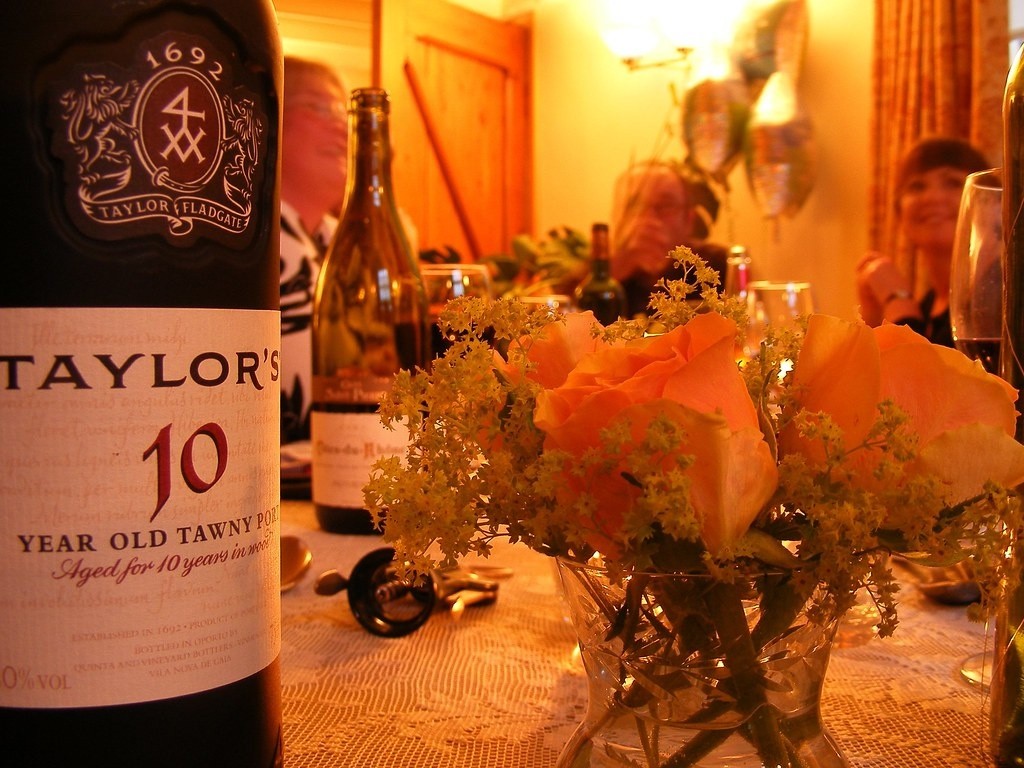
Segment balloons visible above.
[676,0,826,228]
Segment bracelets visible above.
[876,287,917,311]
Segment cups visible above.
[740,281,821,399]
[417,264,495,359]
[511,295,576,345]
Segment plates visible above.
[893,555,997,604]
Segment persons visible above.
[267,51,353,451]
[553,156,739,317]
[849,135,1003,378]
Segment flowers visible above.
[364,243,1024,768]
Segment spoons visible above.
[274,533,312,594]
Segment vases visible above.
[554,540,857,768]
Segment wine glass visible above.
[948,165,1007,689]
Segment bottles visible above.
[718,244,752,298]
[0,0,285,768]
[310,86,431,537]
[573,224,627,325]
[989,45,1024,768]
